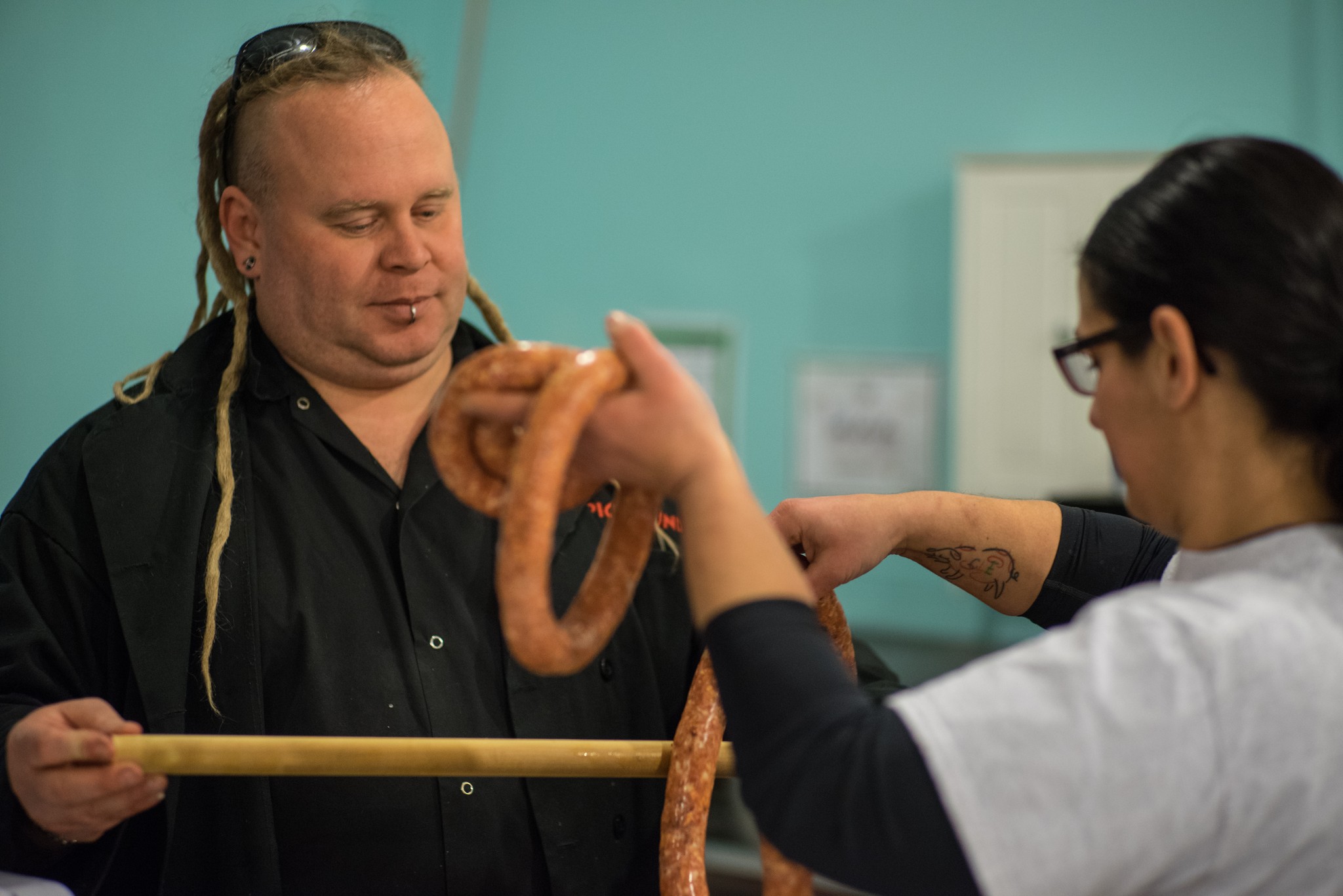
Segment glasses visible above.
[1053,317,1216,396]
[222,21,407,186]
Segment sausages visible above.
[432,340,858,896]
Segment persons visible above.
[0,16,902,896]
[461,136,1343,896]
